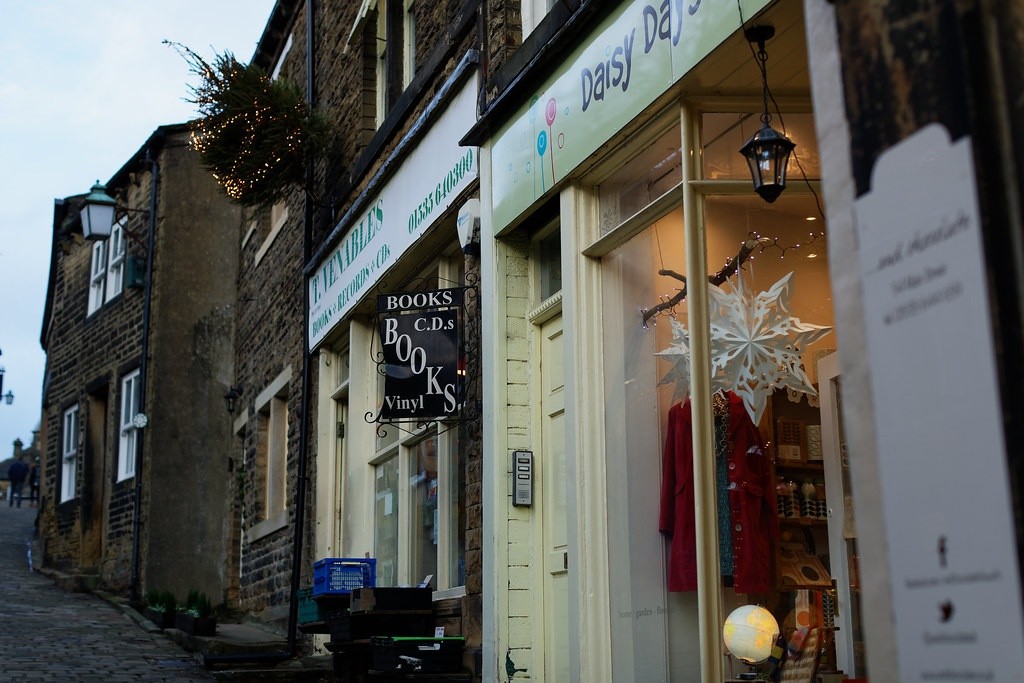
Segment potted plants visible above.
[144,585,216,636]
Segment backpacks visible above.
[34,468,40,486]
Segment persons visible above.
[8,456,41,508]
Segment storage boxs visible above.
[296,558,466,674]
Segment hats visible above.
[35,456,40,461]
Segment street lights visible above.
[78,180,156,601]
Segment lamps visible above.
[224,383,242,416]
[738,24,797,203]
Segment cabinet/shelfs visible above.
[748,383,861,617]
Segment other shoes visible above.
[9,501,14,507]
[17,502,21,508]
[29,502,33,507]
[36,503,40,508]
